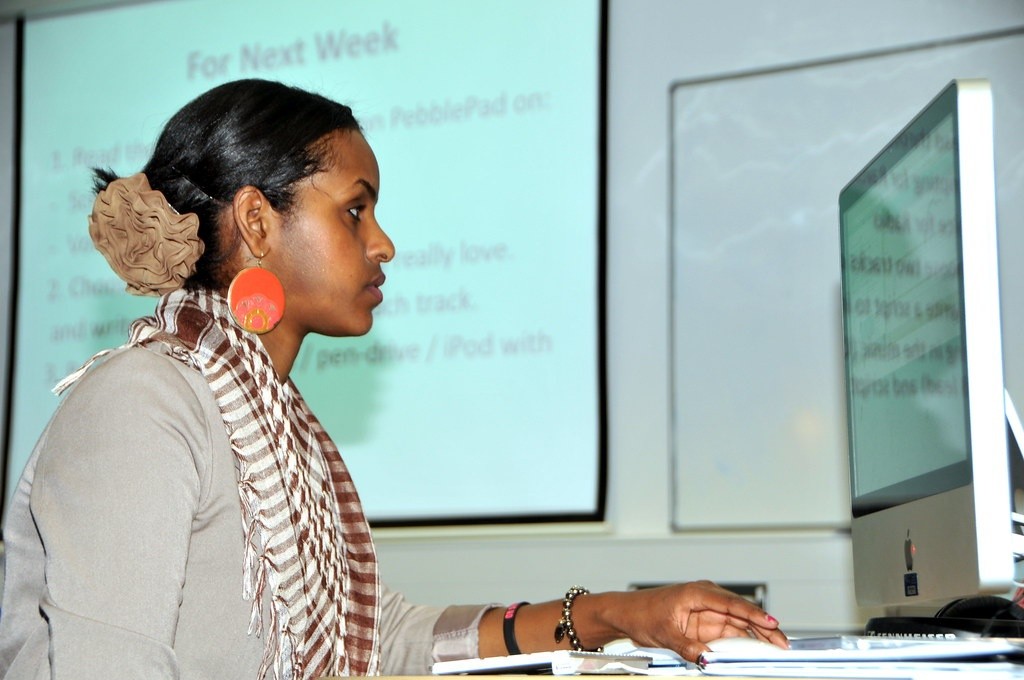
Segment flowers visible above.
[86,173,206,298]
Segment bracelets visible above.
[554,586,603,653]
[503,601,531,655]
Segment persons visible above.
[0,79,789,680]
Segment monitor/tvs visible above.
[838,78,1024,609]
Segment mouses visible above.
[685,637,787,670]
